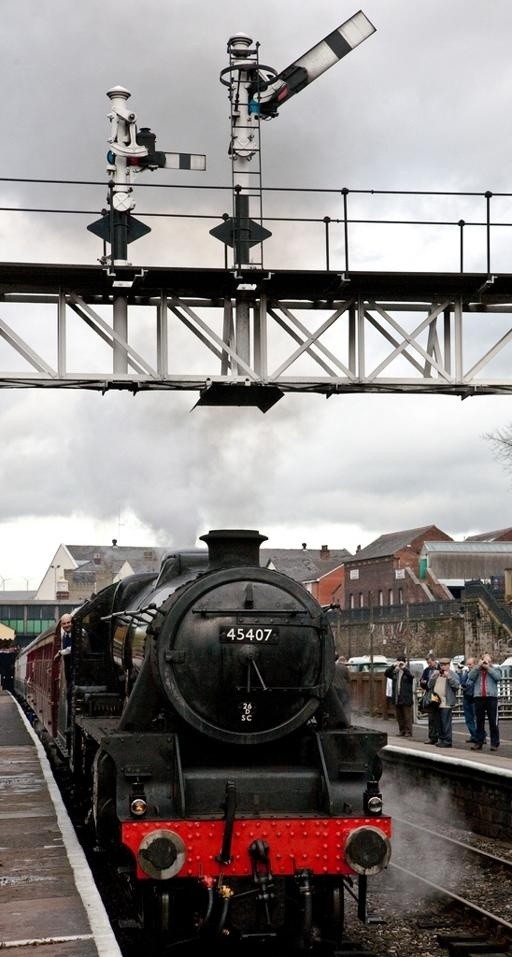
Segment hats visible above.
[440,658,450,663]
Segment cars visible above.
[337,651,512,703]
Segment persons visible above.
[456,656,478,743]
[335,655,352,726]
[427,657,459,748]
[54,611,90,734]
[418,653,442,745]
[466,652,503,751]
[383,654,414,737]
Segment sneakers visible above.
[406,733,412,737]
[396,733,405,736]
[490,746,497,751]
[466,739,476,743]
[471,745,482,750]
[424,739,438,745]
[436,742,452,748]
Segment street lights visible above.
[50,565,62,598]
[11,523,392,955]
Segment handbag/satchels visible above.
[418,692,441,714]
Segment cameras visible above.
[439,670,445,677]
[399,663,403,668]
[480,661,487,672]
